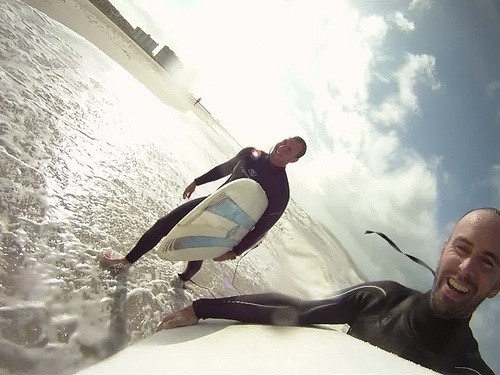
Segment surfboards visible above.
[157,178,269,262]
[72,318,442,375]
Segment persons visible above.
[156,207,500,375]
[99,135,307,290]
